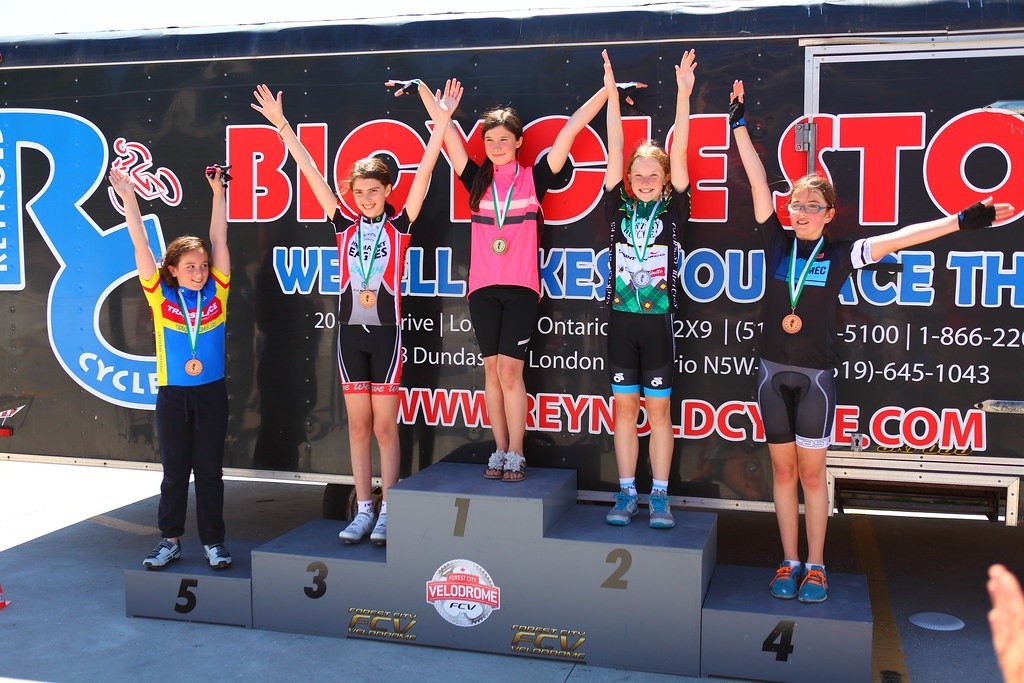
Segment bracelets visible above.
[277,121,289,143]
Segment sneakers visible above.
[203,543,232,566]
[798,565,828,601]
[769,560,802,598]
[338,513,374,542]
[143,538,182,568]
[370,513,387,541]
[605,488,638,525]
[648,491,674,527]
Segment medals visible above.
[781,316,803,333]
[632,270,650,288]
[185,359,202,376]
[491,238,507,255]
[359,291,376,308]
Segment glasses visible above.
[788,203,830,214]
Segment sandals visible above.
[483,449,507,478]
[502,450,526,481]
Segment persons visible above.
[251,77,463,548]
[727,80,1017,598]
[384,79,648,478]
[106,166,231,570]
[978,565,1024,683]
[600,47,697,532]
